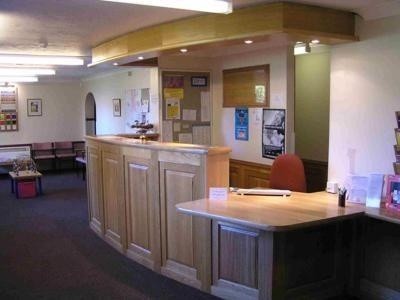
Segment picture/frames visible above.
[112,99,121,117]
[27,99,42,116]
[191,76,208,86]
[386,174,400,212]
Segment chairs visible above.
[31,141,86,180]
[269,153,307,192]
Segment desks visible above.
[360,202,400,300]
[174,187,364,300]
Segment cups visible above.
[338,188,346,208]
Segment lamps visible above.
[293,42,311,55]
[103,0,234,15]
[0,55,84,91]
